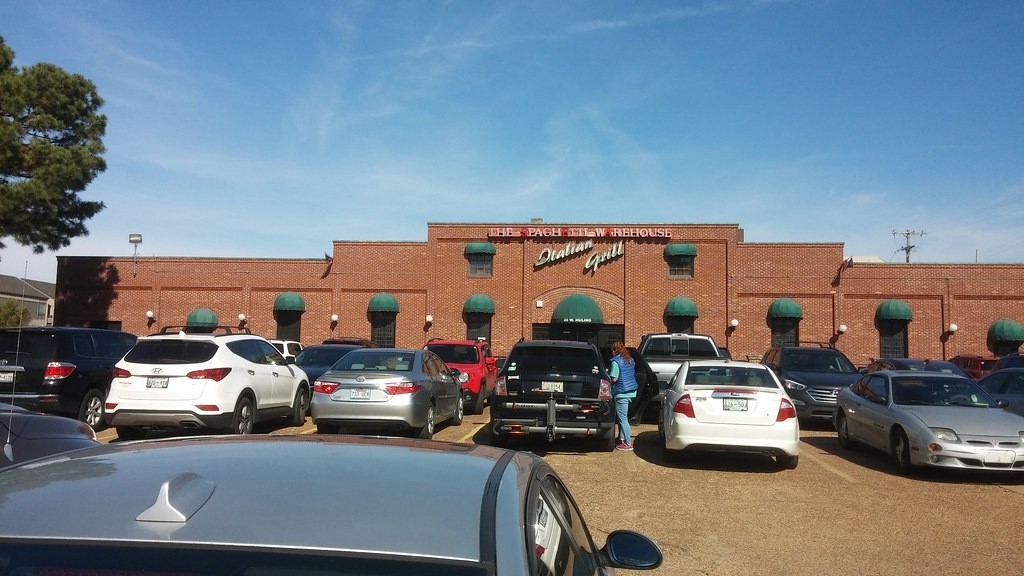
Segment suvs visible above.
[105,324,311,444]
[489,337,660,452]
[758,341,869,424]
[416,338,498,415]
[0,325,140,433]
[266,338,385,400]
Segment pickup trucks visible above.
[634,332,732,405]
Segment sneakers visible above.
[615,437,626,444]
[615,442,634,451]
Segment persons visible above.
[610,342,639,451]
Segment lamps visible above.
[146,310,156,322]
[238,313,247,324]
[837,324,848,335]
[331,314,339,321]
[949,323,957,333]
[425,315,433,323]
[731,319,739,330]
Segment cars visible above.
[832,369,1024,476]
[861,352,1024,413]
[657,359,800,469]
[310,347,466,440]
[1,403,665,575]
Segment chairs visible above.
[695,374,711,384]
[351,363,365,369]
[747,376,762,386]
[650,340,664,353]
[395,364,409,370]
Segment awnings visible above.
[665,296,698,318]
[464,243,496,255]
[368,292,400,313]
[876,299,912,321]
[274,291,305,312]
[551,294,604,324]
[463,294,495,314]
[989,320,1024,342]
[186,308,218,328]
[768,298,803,320]
[664,244,697,257]
